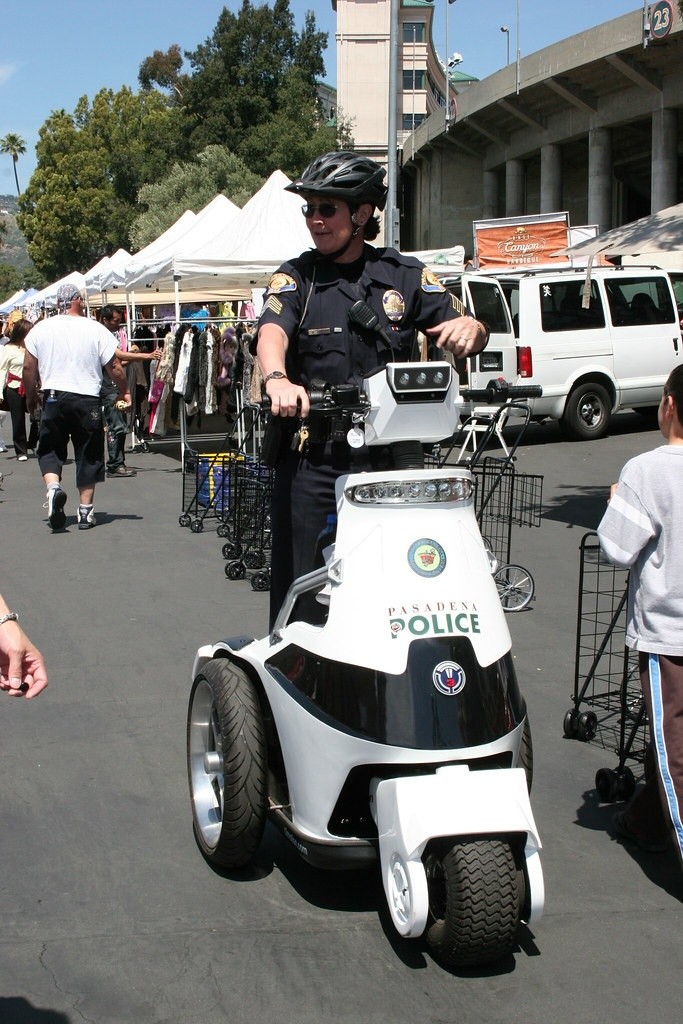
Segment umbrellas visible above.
[548,202,683,308]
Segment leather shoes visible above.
[107,466,137,478]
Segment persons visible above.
[99,304,163,478]
[21,283,132,534]
[249,152,490,632]
[597,364,683,868]
[0,319,40,461]
[0,594,48,699]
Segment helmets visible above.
[284,151,389,212]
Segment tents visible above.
[125,168,470,475]
[0,168,242,350]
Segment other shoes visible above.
[17,453,27,461]
[613,810,667,852]
[32,447,38,457]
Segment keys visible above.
[291,430,309,454]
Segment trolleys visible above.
[561,529,652,804]
[177,402,538,615]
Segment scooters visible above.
[183,358,548,975]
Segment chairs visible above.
[561,293,664,325]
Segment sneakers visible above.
[42,487,67,533]
[77,504,96,529]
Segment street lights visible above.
[500,26,510,65]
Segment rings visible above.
[30,407,33,409]
[460,337,467,341]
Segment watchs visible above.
[264,371,287,384]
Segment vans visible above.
[426,262,683,441]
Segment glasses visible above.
[301,201,361,218]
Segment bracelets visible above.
[123,393,129,394]
[471,318,483,335]
[0,613,18,625]
